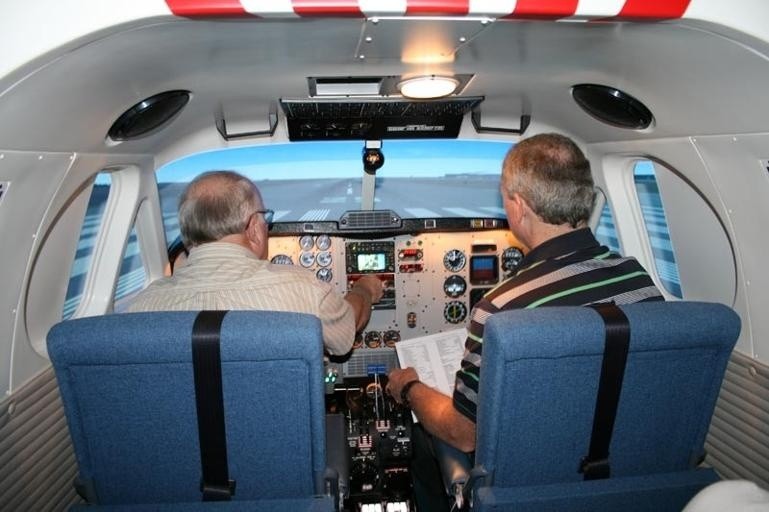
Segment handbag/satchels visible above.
[350,283,374,304]
[399,379,420,408]
[388,326,470,425]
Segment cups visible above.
[244,207,275,232]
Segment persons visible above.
[121,169,387,362]
[381,132,669,464]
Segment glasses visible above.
[394,76,459,99]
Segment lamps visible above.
[430,301,741,512]
[47,310,351,512]
